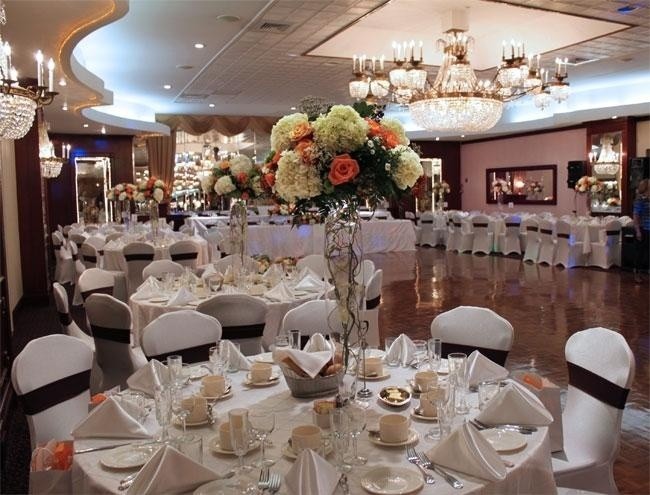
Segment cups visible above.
[121,392,144,426]
[153,263,300,299]
[479,379,502,410]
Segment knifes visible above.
[74,443,129,454]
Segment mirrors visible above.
[590,130,623,214]
[485,164,557,205]
[74,155,112,225]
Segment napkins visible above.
[381,331,419,366]
[283,446,343,493]
[466,347,510,386]
[68,393,158,440]
[123,442,220,495]
[216,338,253,374]
[474,378,555,427]
[130,266,330,306]
[426,423,509,486]
[126,357,172,398]
[301,331,332,352]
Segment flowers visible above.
[102,184,137,202]
[196,151,272,214]
[574,175,601,195]
[525,176,545,193]
[431,182,451,202]
[131,175,172,207]
[261,90,426,231]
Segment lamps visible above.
[344,5,571,138]
[0,0,59,139]
[590,131,622,177]
[36,100,72,181]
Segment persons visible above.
[633,179,650,284]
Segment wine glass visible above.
[145,328,474,475]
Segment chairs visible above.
[77,267,116,393]
[123,241,155,302]
[139,306,224,367]
[276,297,353,350]
[82,291,147,402]
[193,293,269,355]
[429,303,516,372]
[67,240,89,307]
[49,281,97,353]
[46,205,639,284]
[10,336,95,492]
[351,257,385,351]
[547,325,638,493]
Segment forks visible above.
[401,442,464,490]
[255,464,282,495]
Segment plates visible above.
[98,446,155,470]
[191,475,251,495]
[363,467,423,495]
[291,291,308,296]
[476,429,527,454]
